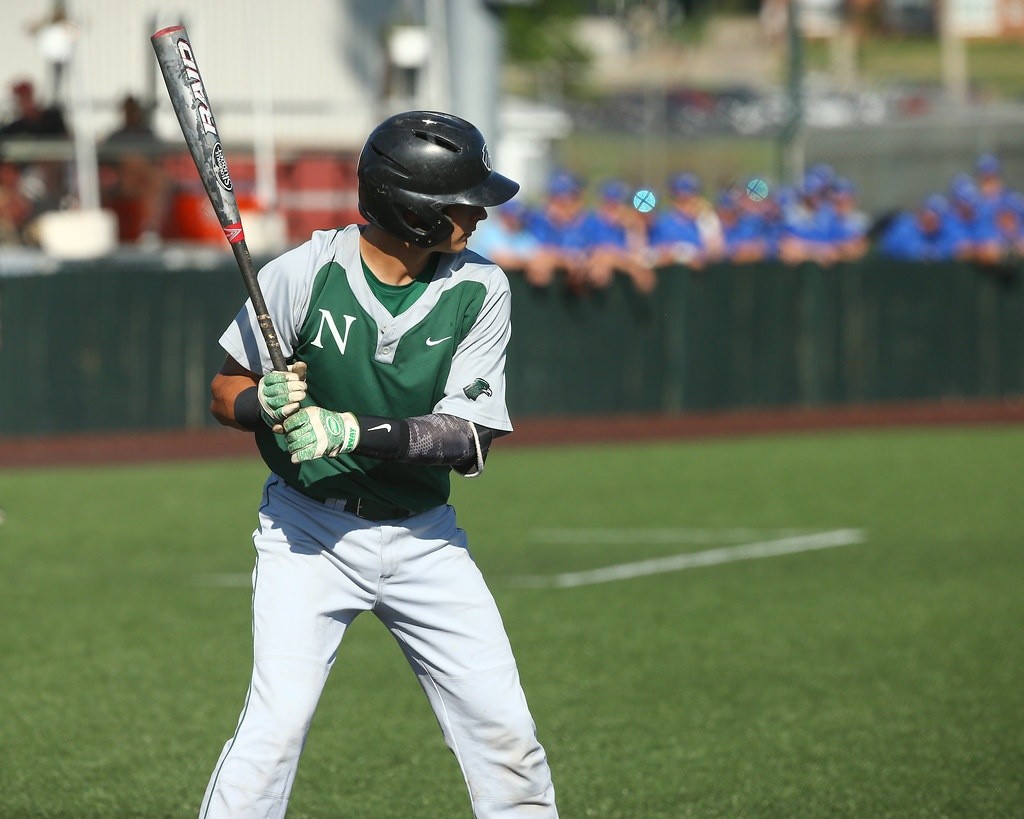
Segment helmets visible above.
[358,111,520,248]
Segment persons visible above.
[468,151,1024,298]
[197,109,560,819]
[0,83,169,237]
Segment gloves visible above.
[273,406,361,464]
[258,362,307,427]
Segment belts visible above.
[286,481,409,518]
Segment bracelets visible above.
[350,411,410,463]
[233,384,261,433]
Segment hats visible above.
[926,195,950,214]
[549,172,579,194]
[803,173,825,195]
[776,188,799,208]
[835,175,854,192]
[669,174,698,192]
[977,156,1002,173]
[602,180,629,200]
[953,176,977,204]
[815,165,835,184]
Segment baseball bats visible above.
[149,25,288,373]
